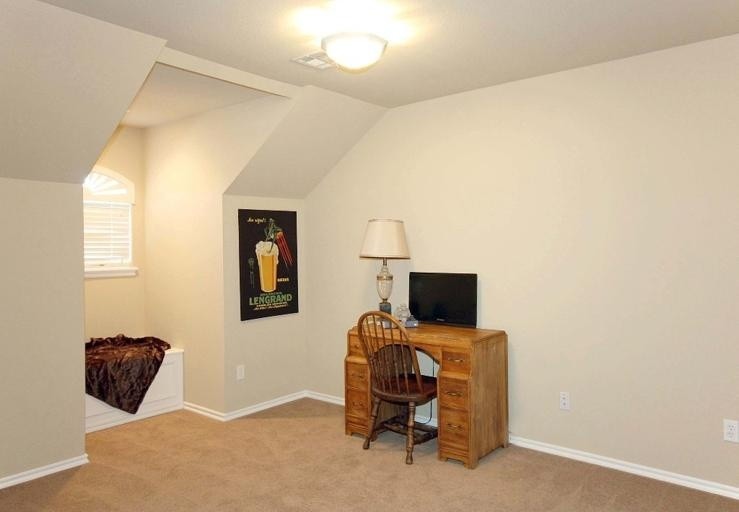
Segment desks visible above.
[344,328,509,470]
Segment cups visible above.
[255,242,278,292]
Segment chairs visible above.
[357,311,437,464]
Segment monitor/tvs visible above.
[408,271,477,329]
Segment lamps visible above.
[359,218,411,329]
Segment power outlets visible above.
[723,418,739,443]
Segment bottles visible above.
[393,304,416,323]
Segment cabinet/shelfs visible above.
[85,348,185,438]
